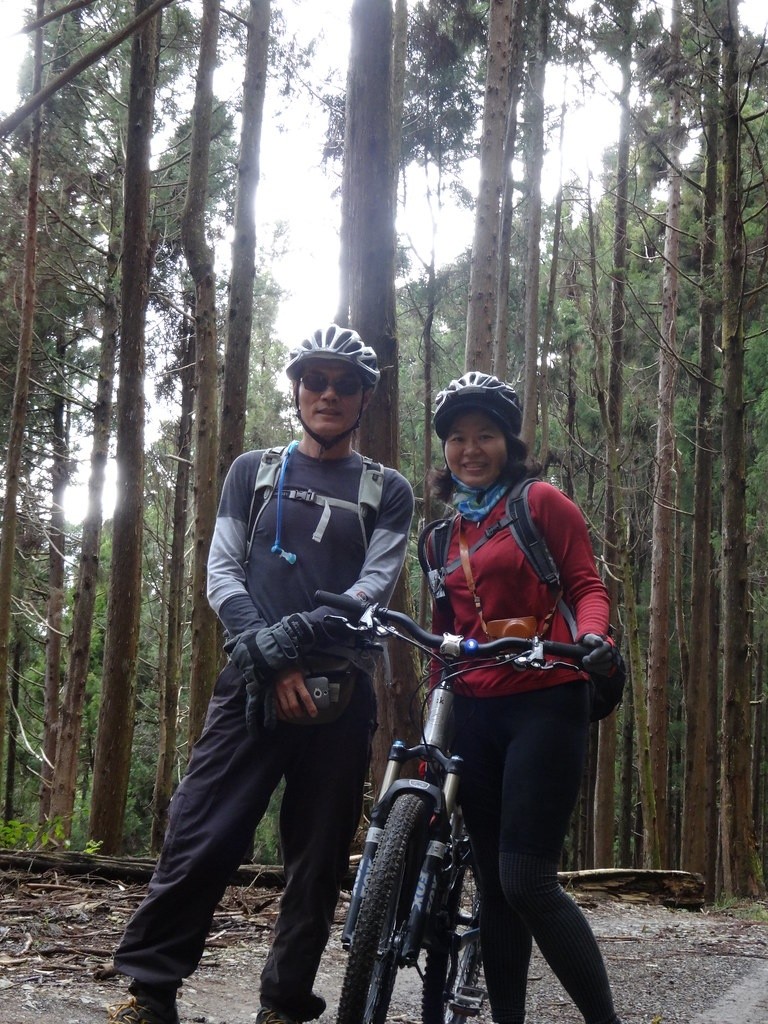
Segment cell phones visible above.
[295,677,330,710]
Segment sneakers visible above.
[108,996,179,1023]
[256,1006,305,1024]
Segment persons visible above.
[106,323,415,1024]
[418,371,622,1024]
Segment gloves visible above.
[223,612,317,740]
[579,631,622,676]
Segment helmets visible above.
[430,372,523,440]
[282,323,381,395]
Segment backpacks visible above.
[589,653,629,721]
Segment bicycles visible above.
[314,590,591,1024]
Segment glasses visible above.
[298,367,367,394]
